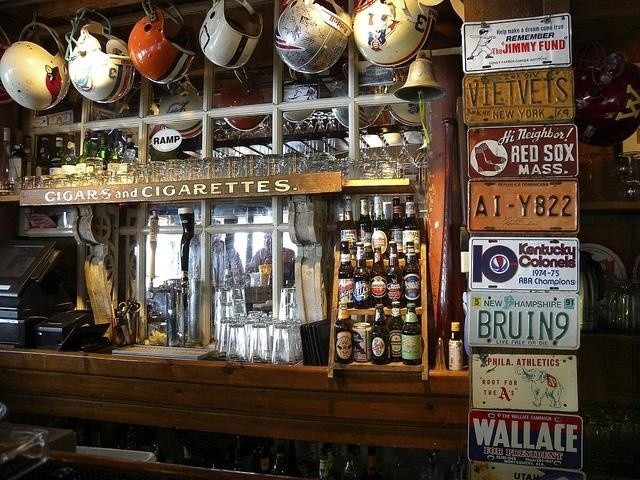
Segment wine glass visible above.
[579,152,603,203]
[212,111,335,141]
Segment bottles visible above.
[70,421,465,480]
[336,197,422,311]
[334,303,354,364]
[446,320,464,374]
[6,127,137,191]
[370,302,422,365]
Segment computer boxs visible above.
[0,307,74,349]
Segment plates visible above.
[580,244,626,277]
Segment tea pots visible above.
[580,250,605,333]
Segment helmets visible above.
[274,0,439,75]
[0,0,264,111]
[218,68,425,131]
[119,75,203,140]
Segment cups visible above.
[168,291,186,349]
[139,150,399,192]
[110,317,137,349]
[258,264,272,286]
[213,286,302,365]
[617,150,640,201]
[578,404,639,471]
[605,278,640,335]
[250,272,261,286]
[147,322,167,346]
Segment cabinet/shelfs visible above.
[327,242,428,379]
[569,1,640,480]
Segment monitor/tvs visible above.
[0,236,77,307]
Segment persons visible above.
[188,220,243,282]
[249,231,295,275]
